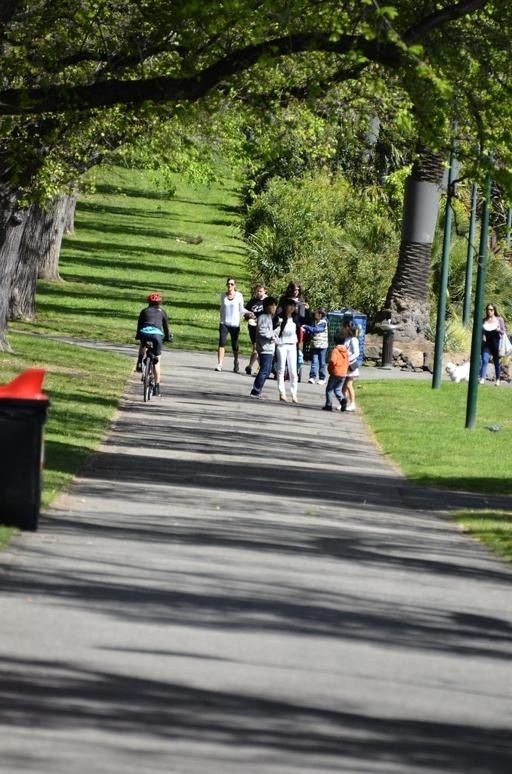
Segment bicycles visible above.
[134,336,172,402]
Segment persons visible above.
[322,333,351,413]
[137,292,170,396]
[215,277,254,373]
[340,320,360,412]
[479,301,506,386]
[250,282,329,405]
[245,284,271,375]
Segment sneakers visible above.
[494,379,500,386]
[233,359,240,371]
[135,359,144,373]
[152,383,160,396]
[291,394,299,402]
[279,393,288,403]
[322,405,332,410]
[244,366,252,375]
[316,379,327,385]
[346,402,356,411]
[307,377,315,384]
[214,363,224,371]
[336,399,350,412]
[250,392,268,400]
[477,378,485,385]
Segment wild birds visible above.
[483,425,504,432]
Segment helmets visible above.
[147,291,161,303]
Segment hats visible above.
[287,282,300,290]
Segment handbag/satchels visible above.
[496,332,512,357]
[349,358,359,371]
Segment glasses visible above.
[227,282,235,286]
[486,307,494,312]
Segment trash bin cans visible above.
[326,307,367,367]
[0,368,50,531]
